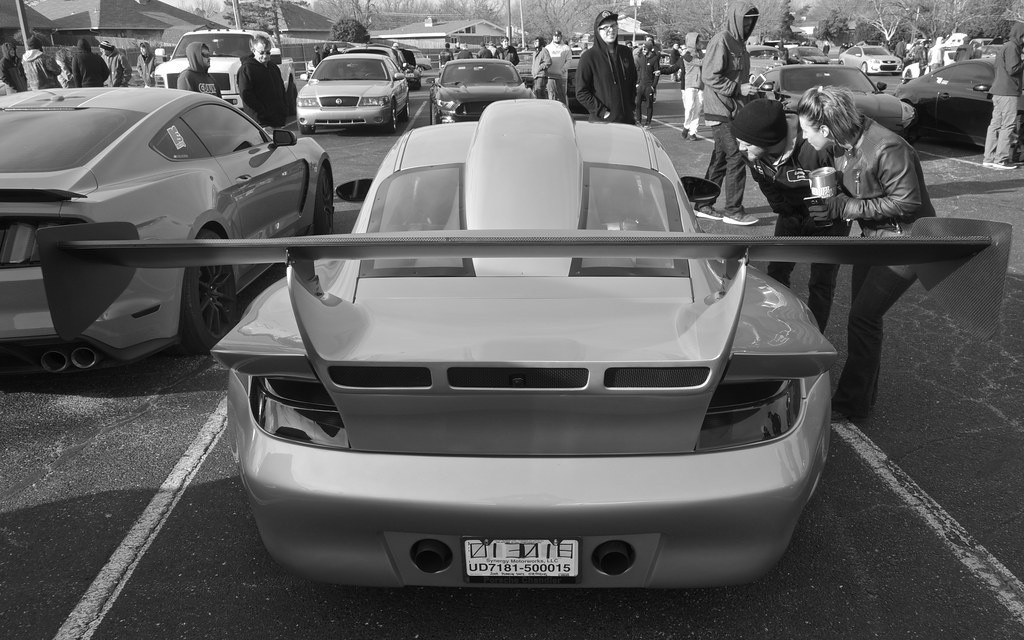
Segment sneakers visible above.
[993,161,1017,170]
[693,204,723,220]
[983,158,994,166]
[722,206,758,225]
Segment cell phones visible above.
[803,196,834,227]
[751,73,766,88]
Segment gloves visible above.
[808,185,849,221]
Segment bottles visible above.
[809,167,836,198]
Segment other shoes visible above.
[681,127,689,139]
[690,134,698,141]
[644,122,650,127]
[831,404,869,423]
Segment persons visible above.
[477,42,493,58]
[22,36,61,90]
[313,45,320,69]
[802,41,830,56]
[983,23,1024,169]
[545,31,572,105]
[797,86,934,425]
[841,42,854,50]
[733,99,853,333]
[137,42,160,88]
[532,37,552,98]
[0,43,26,94]
[440,43,454,66]
[896,38,975,77]
[454,42,462,53]
[100,41,132,87]
[689,1,759,226]
[237,35,288,132]
[493,37,519,66]
[177,42,222,98]
[457,45,474,59]
[625,31,704,140]
[564,41,568,45]
[575,10,636,125]
[778,40,799,64]
[322,43,341,59]
[55,39,109,87]
[392,43,405,64]
[488,41,497,56]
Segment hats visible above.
[644,41,653,47]
[99,41,115,51]
[27,36,41,49]
[729,99,788,146]
[597,11,618,26]
[552,30,562,37]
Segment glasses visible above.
[598,25,619,30]
[203,51,211,57]
[255,49,270,55]
